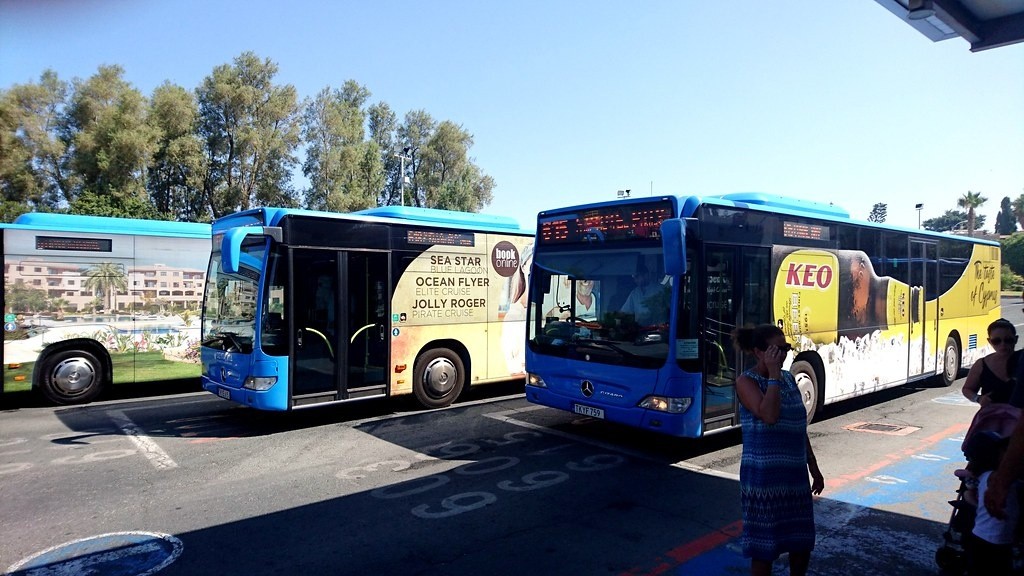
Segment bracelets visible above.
[974,395,980,402]
[767,378,780,385]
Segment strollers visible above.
[934,391,1024,572]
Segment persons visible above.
[499,241,600,375]
[962,317,1024,576]
[734,324,824,576]
[614,264,668,341]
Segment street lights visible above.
[394,145,413,207]
[915,204,923,230]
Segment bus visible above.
[199,207,599,412]
[524,192,1002,439]
[0,212,285,407]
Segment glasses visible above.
[776,343,791,352]
[991,336,1016,345]
